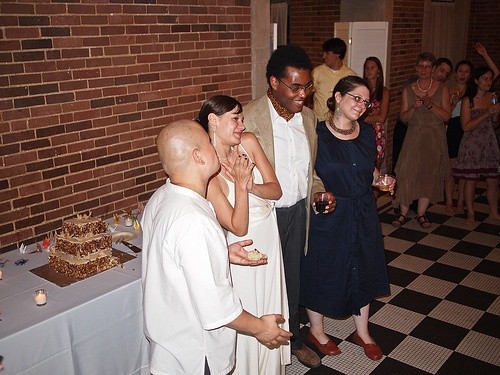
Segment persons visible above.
[392,57,454,214]
[139,118,293,375]
[392,52,451,228]
[311,38,360,122]
[299,75,396,361]
[445,42,500,214]
[192,95,292,375]
[450,66,500,228]
[242,44,337,368]
[362,56,391,199]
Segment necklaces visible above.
[329,116,357,135]
[222,146,234,160]
[416,79,433,92]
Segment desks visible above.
[0,213,150,375]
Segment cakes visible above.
[48,210,113,279]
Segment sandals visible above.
[417,215,431,228]
[394,215,407,227]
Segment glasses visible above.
[276,77,314,92]
[344,92,371,108]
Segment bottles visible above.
[491,92,497,105]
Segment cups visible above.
[314,192,329,213]
[0,270,3,280]
[33,288,47,306]
[378,173,394,191]
[124,215,133,227]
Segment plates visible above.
[112,232,136,242]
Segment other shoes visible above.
[291,342,321,368]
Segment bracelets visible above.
[427,103,434,109]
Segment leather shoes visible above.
[352,329,382,360]
[307,328,342,355]
